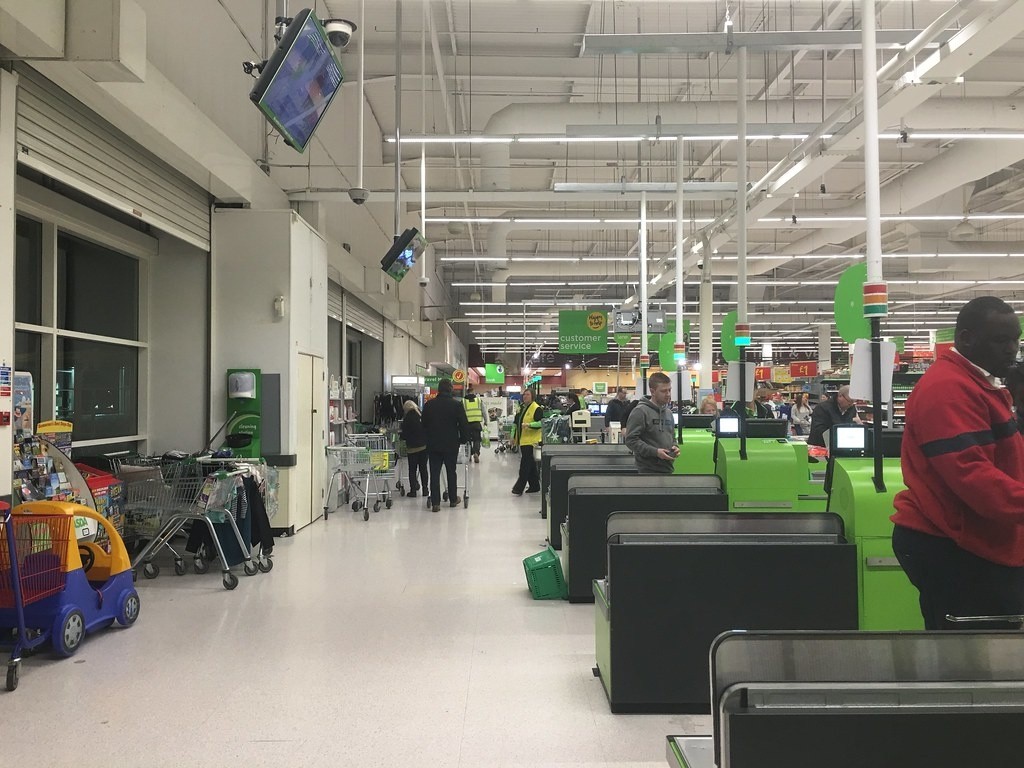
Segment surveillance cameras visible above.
[348,187,369,205]
[417,277,429,287]
[697,260,704,269]
[325,19,353,47]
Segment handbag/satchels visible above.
[800,420,811,434]
[482,425,491,448]
[395,439,408,457]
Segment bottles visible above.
[892,384,914,390]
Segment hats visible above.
[438,379,453,392]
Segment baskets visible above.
[522,546,569,600]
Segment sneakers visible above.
[432,505,440,512]
[450,496,461,507]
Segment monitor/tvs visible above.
[671,411,679,427]
[682,414,714,429]
[869,428,904,458]
[716,416,740,438]
[600,405,608,416]
[588,404,600,416]
[745,418,788,439]
[833,423,868,455]
[380,227,429,282]
[251,8,345,153]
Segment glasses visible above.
[621,392,627,394]
[842,394,854,405]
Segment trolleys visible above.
[426,439,470,509]
[104,448,275,589]
[322,433,421,522]
[0,507,75,693]
[494,415,519,453]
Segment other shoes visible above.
[407,492,416,496]
[423,491,429,495]
[511,490,522,495]
[473,453,479,463]
[525,489,540,493]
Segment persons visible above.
[822,393,830,401]
[889,296,1024,630]
[398,399,428,497]
[510,389,543,496]
[752,388,793,421]
[548,392,580,415]
[605,387,628,428]
[701,399,717,416]
[421,378,470,510]
[625,373,680,473]
[578,388,588,410]
[461,388,490,464]
[791,394,812,436]
[808,386,862,448]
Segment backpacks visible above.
[556,418,570,437]
